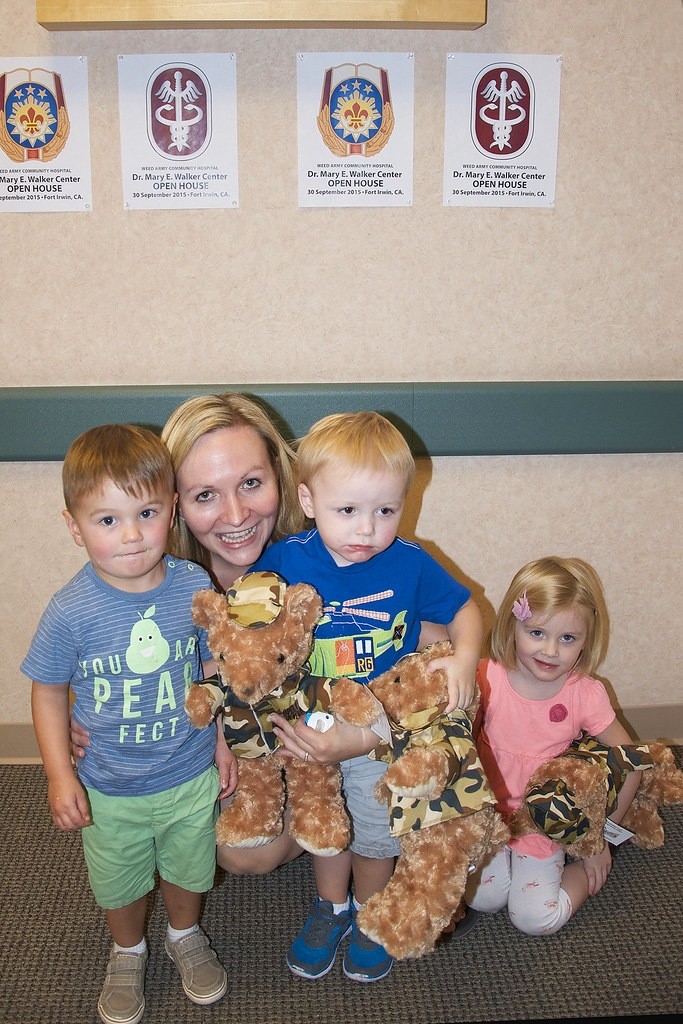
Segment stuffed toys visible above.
[508,738,683,858]
[355,640,509,959]
[187,572,377,859]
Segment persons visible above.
[464,555,642,937]
[21,423,238,1023]
[248,411,483,983]
[67,395,455,876]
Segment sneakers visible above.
[343,904,395,980]
[288,896,353,978]
[99,942,149,1024]
[165,924,228,1006]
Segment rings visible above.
[305,752,308,762]
